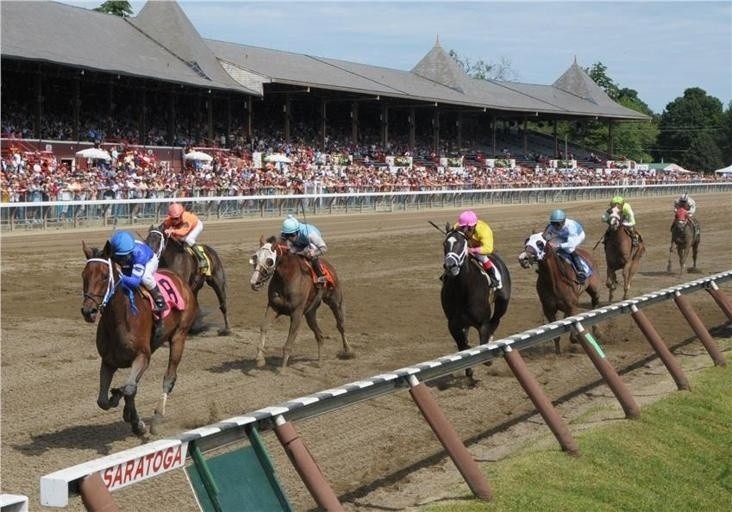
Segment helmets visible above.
[459,211,478,227]
[548,209,566,223]
[611,195,626,207]
[110,231,137,254]
[680,192,689,202]
[167,203,184,220]
[281,214,302,235]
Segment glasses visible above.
[284,233,299,237]
[170,217,181,220]
[461,226,474,231]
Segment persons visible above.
[447,139,732,191]
[1,83,446,226]
[601,195,638,245]
[161,203,209,268]
[110,230,165,308]
[670,193,696,232]
[544,209,586,276]
[453,210,499,286]
[281,215,335,290]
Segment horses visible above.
[440,221,511,386]
[604,200,646,306]
[80,239,196,438]
[667,201,701,278]
[518,227,601,355]
[145,224,230,334]
[249,235,354,376]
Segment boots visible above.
[149,285,164,308]
[570,251,585,280]
[486,267,499,287]
[310,260,325,282]
[190,244,209,270]
[631,229,639,246]
